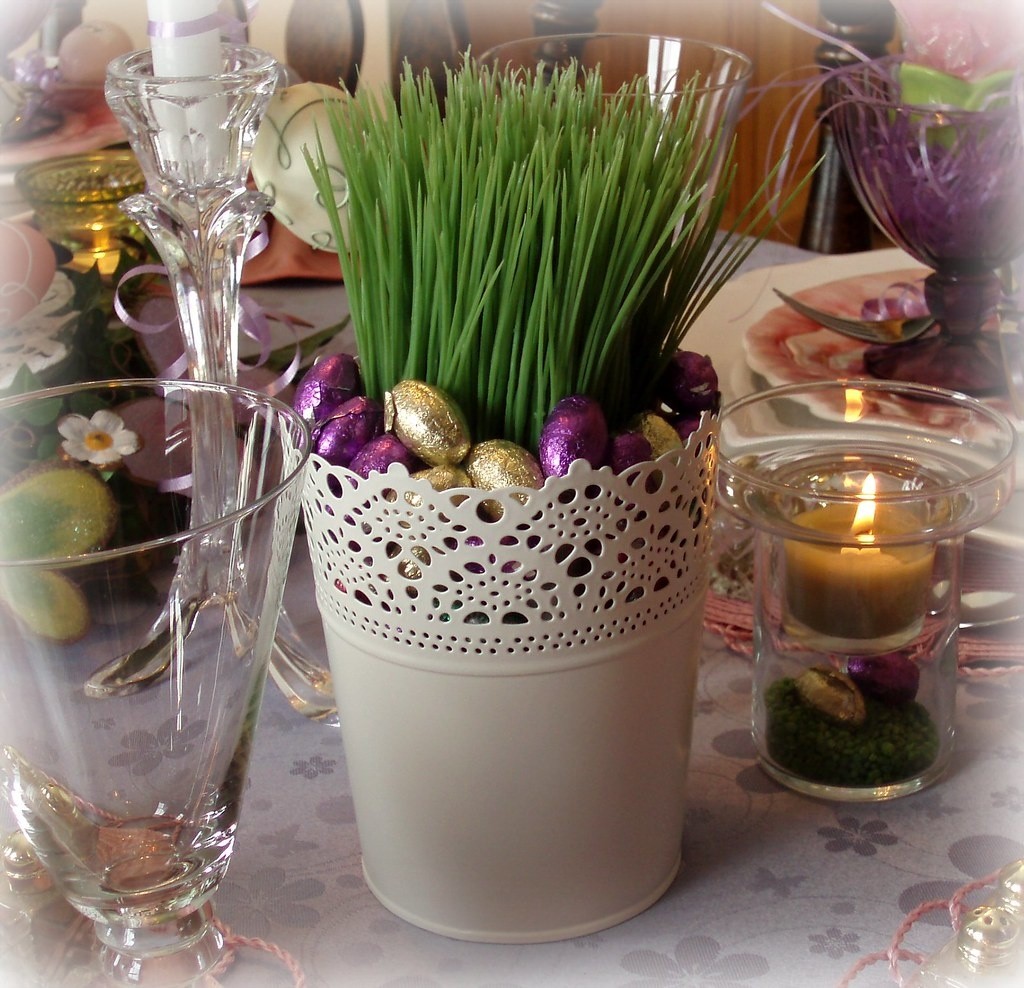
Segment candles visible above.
[146,0,230,187]
[712,381,1014,801]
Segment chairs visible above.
[285,0,365,98]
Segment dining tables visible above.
[0,54,1024,988]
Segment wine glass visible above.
[0,377,311,987]
[822,55,1024,405]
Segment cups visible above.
[475,31,754,357]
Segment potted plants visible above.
[276,45,832,944]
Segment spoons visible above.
[957,589,1023,631]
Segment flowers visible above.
[58,410,145,466]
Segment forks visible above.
[773,288,936,344]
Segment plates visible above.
[722,269,1024,563]
[0,104,69,142]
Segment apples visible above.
[59,20,133,84]
